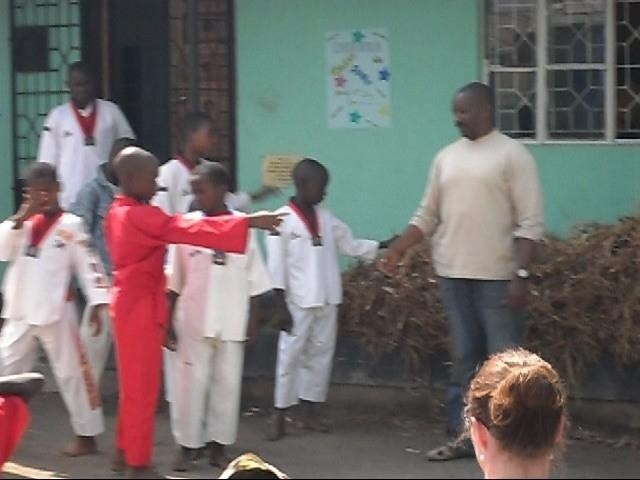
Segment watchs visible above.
[514,267,534,282]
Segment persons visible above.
[68,136,139,388]
[378,79,544,462]
[148,114,255,217]
[160,161,255,471]
[102,146,290,480]
[1,161,112,457]
[1,371,46,469]
[462,348,568,479]
[36,64,136,212]
[264,158,400,443]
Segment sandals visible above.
[426,441,467,461]
